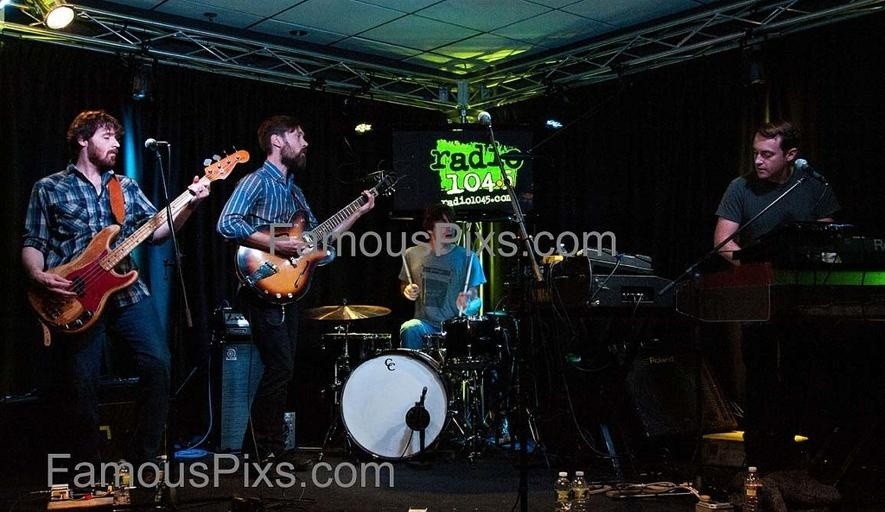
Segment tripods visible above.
[317,357,364,466]
[485,397,551,473]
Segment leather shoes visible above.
[234,461,300,489]
[271,453,314,474]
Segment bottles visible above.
[743,467,762,511]
[553,472,572,511]
[571,471,589,511]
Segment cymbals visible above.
[305,305,392,321]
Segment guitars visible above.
[234,169,399,305]
[26,145,250,333]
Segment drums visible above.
[440,314,501,370]
[339,348,455,461]
[422,333,447,364]
[324,332,393,403]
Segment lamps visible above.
[28,0,73,29]
[354,105,372,135]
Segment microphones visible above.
[144,138,171,151]
[477,111,492,130]
[794,158,829,187]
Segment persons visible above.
[215,114,375,455]
[396,204,488,353]
[23,111,211,497]
[715,121,843,508]
[501,187,546,280]
